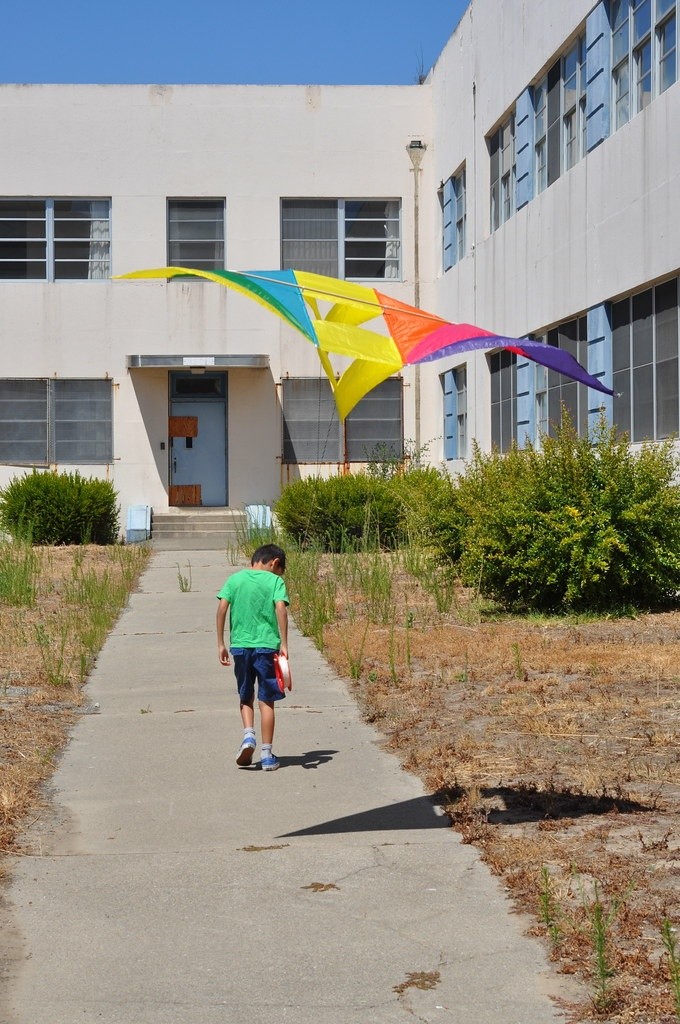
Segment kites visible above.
[110,266,623,423]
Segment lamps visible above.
[190,367,206,374]
[411,141,423,148]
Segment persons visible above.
[216,543,291,773]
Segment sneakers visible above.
[261,754,280,770]
[236,738,256,766]
